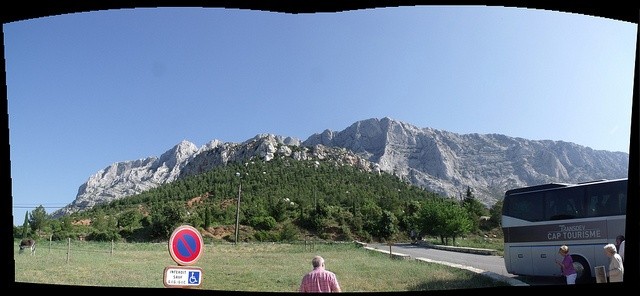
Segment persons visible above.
[595,243,624,283]
[299,256,342,293]
[557,245,578,285]
[616,235,626,254]
[410,228,422,240]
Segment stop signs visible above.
[169,225,203,265]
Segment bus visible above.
[502,178,627,284]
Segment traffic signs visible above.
[164,266,202,288]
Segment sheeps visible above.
[18,238,37,258]
